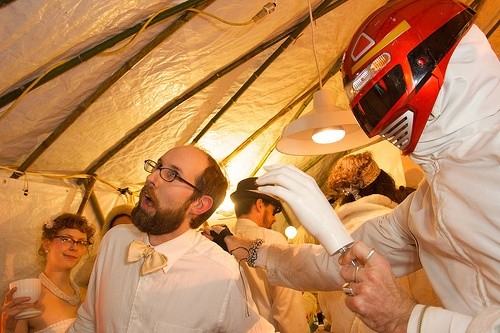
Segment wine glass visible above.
[8,278,41,319]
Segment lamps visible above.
[276,0,382,157]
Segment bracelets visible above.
[245,239,265,267]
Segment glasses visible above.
[144,159,215,206]
[50,235,93,248]
[272,206,280,215]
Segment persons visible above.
[230,177,310,333]
[74,204,133,288]
[66,145,275,333]
[317,153,443,333]
[204,0,500,333]
[0,213,95,333]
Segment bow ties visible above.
[125,240,168,276]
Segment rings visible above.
[365,247,375,262]
[343,281,355,296]
[356,265,361,283]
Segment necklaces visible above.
[39,272,81,305]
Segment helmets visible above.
[340,0,478,158]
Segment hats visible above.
[102,204,133,236]
[230,175,283,212]
